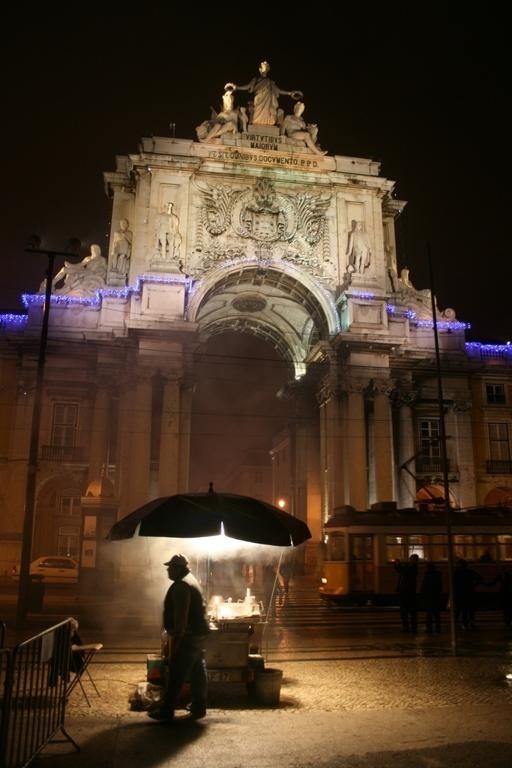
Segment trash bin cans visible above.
[28,574,45,614]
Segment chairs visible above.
[63,617,104,708]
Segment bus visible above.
[314,495,512,612]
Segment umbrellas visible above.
[104,481,313,547]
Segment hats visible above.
[163,553,189,568]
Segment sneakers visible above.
[147,702,206,721]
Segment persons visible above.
[420,562,443,633]
[394,553,418,636]
[195,60,329,155]
[343,221,372,274]
[156,202,183,260]
[260,565,282,622]
[396,269,436,309]
[450,557,484,629]
[147,553,210,721]
[36,216,132,298]
[487,563,512,632]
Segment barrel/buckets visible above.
[255,670,283,706]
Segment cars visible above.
[10,554,78,590]
